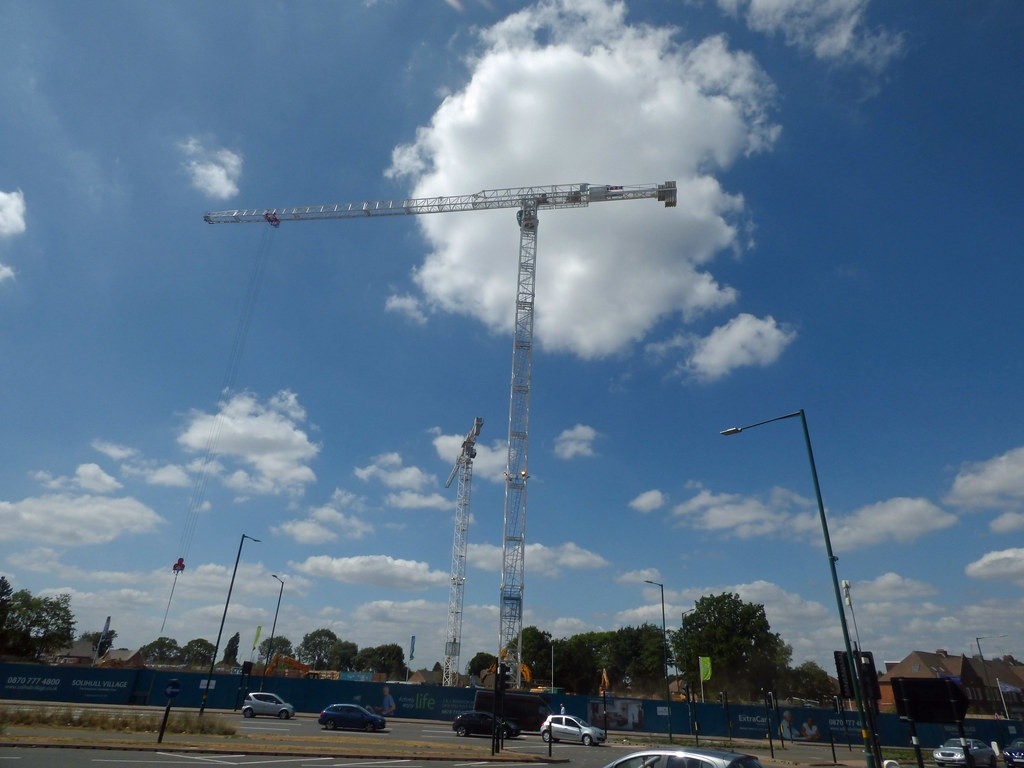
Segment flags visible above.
[997,679,1022,693]
[700,657,712,680]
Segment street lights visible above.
[719,409,883,768]
[976,634,1009,747]
[197,533,262,716]
[644,577,673,739]
[266,571,283,694]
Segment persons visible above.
[777,711,821,741]
[373,686,396,717]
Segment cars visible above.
[541,712,602,747]
[932,738,995,768]
[1002,736,1024,768]
[600,742,764,768]
[244,690,297,718]
[317,704,384,731]
[453,708,520,738]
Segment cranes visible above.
[441,414,484,686]
[201,179,680,690]
